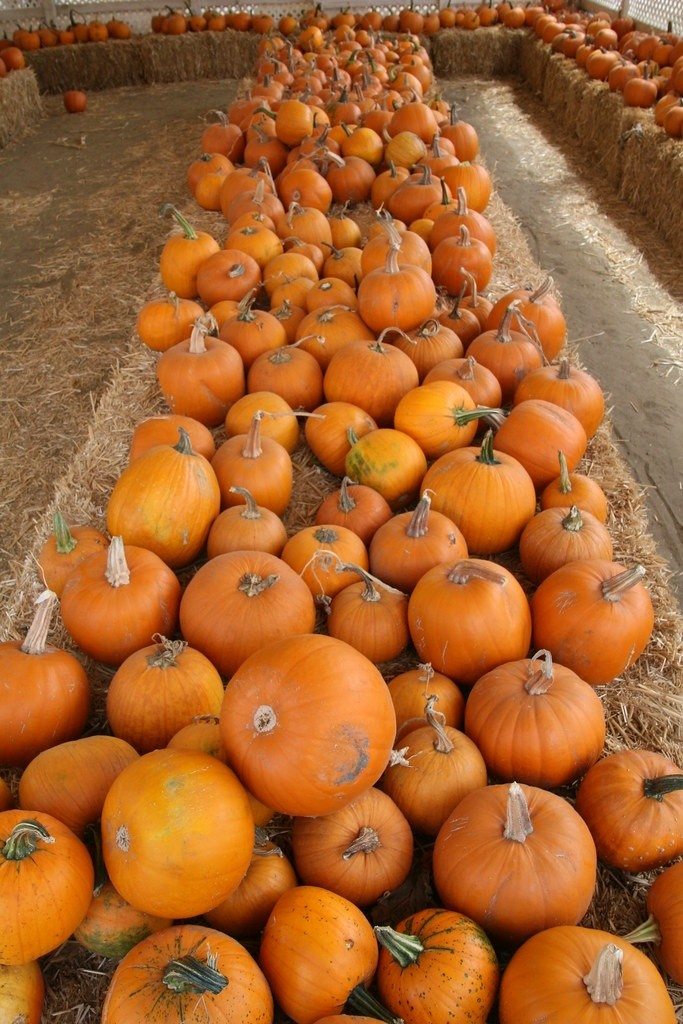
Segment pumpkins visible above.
[0,0,683,1024]
